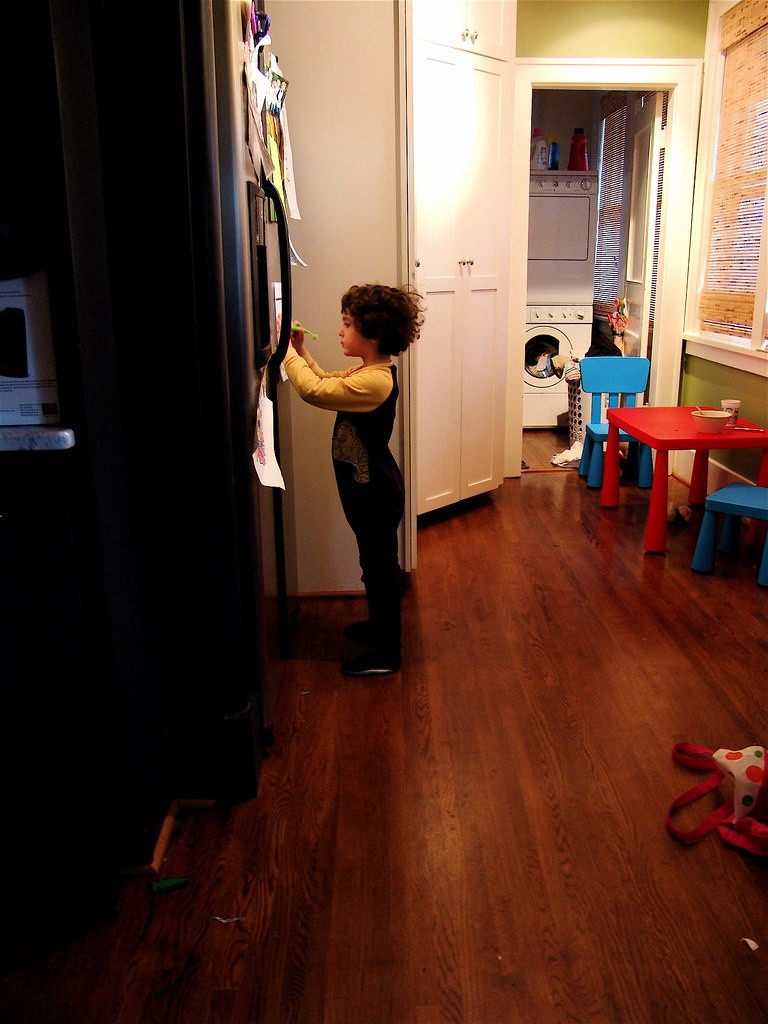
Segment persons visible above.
[276,283,422,678]
[269,75,288,115]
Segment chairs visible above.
[689,482,768,585]
[577,357,653,489]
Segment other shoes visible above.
[345,621,374,643]
[342,657,395,674]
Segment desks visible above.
[599,406,768,553]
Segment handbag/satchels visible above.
[666,743,768,856]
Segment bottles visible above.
[548,139,560,170]
[567,127,590,171]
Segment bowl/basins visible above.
[691,410,731,434]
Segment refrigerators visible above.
[0,0,300,817]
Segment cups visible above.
[721,399,741,426]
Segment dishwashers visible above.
[526,176,599,306]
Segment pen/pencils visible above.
[730,427,765,432]
[291,325,319,340]
